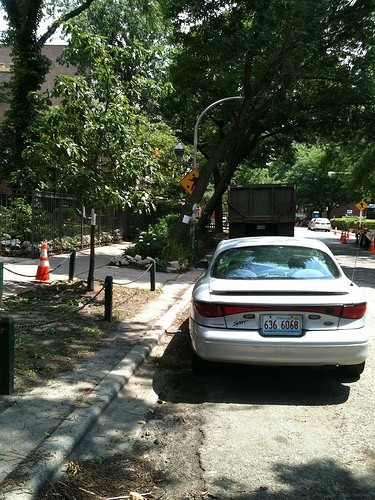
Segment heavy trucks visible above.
[226,182,298,240]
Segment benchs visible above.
[227,269,324,277]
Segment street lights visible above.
[328,171,364,228]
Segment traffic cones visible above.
[338,229,350,244]
[367,236,375,253]
[30,243,53,284]
[333,226,338,236]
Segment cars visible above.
[307,217,332,232]
[294,212,306,223]
[189,235,370,376]
